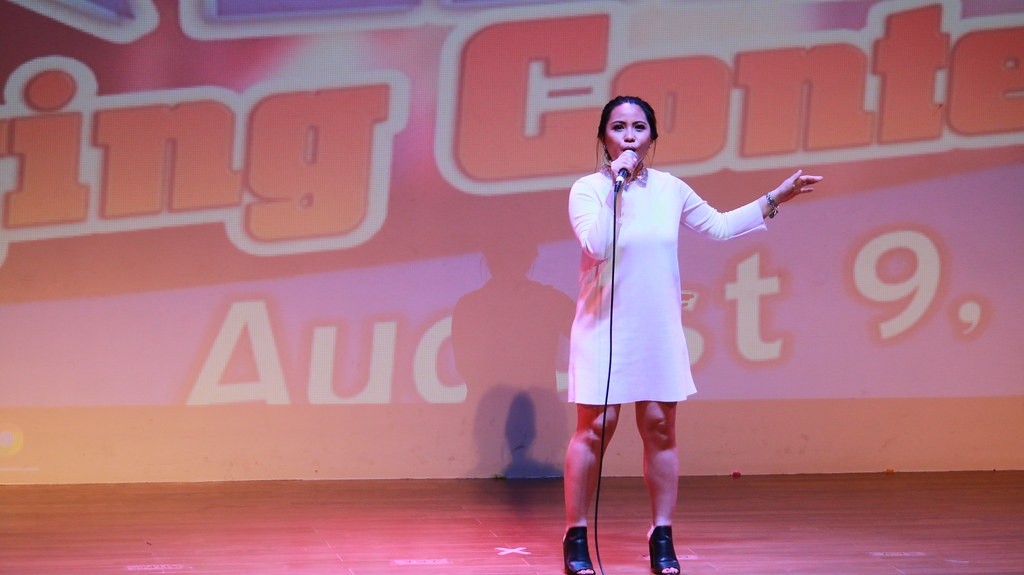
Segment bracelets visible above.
[766,193,779,219]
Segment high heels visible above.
[649,525,680,575]
[562,526,595,575]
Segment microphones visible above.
[615,150,637,192]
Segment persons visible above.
[562,96,823,575]
